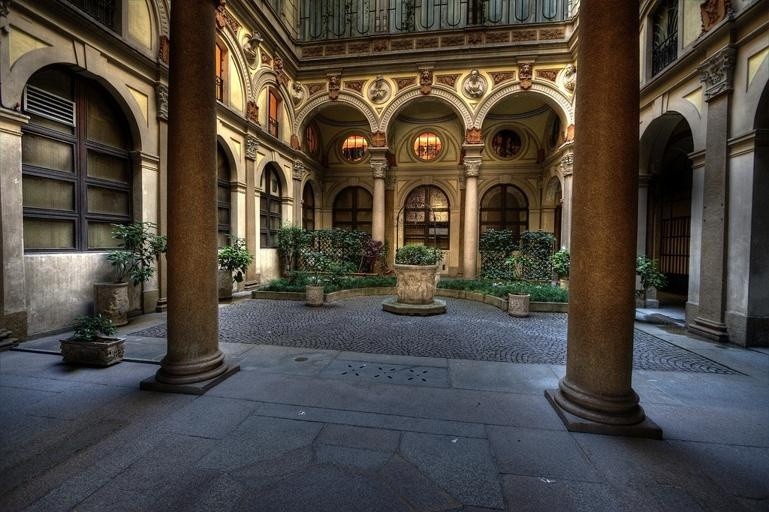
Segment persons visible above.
[466,70,483,97]
[563,64,576,91]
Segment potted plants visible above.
[59,219,168,367]
[304,254,331,306]
[505,255,531,317]
[392,245,441,303]
[217,233,254,301]
